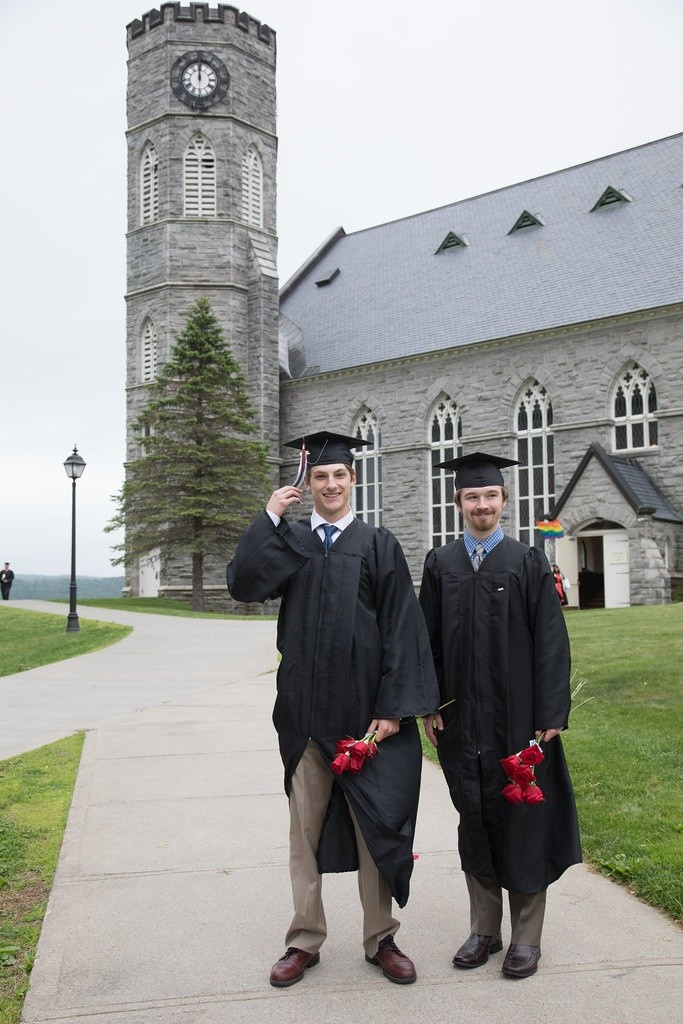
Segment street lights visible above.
[63,443,85,633]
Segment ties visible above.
[472,543,484,571]
[320,524,338,553]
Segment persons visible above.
[550,560,570,606]
[420,450,581,980]
[0,562,14,599]
[228,431,419,986]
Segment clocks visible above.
[169,50,231,109]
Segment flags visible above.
[536,519,567,539]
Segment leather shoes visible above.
[453,933,503,967]
[269,947,320,986]
[365,933,417,984]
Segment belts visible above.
[501,943,540,977]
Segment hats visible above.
[432,452,522,486]
[283,431,374,505]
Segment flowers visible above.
[498,667,595,809]
[329,699,456,778]
